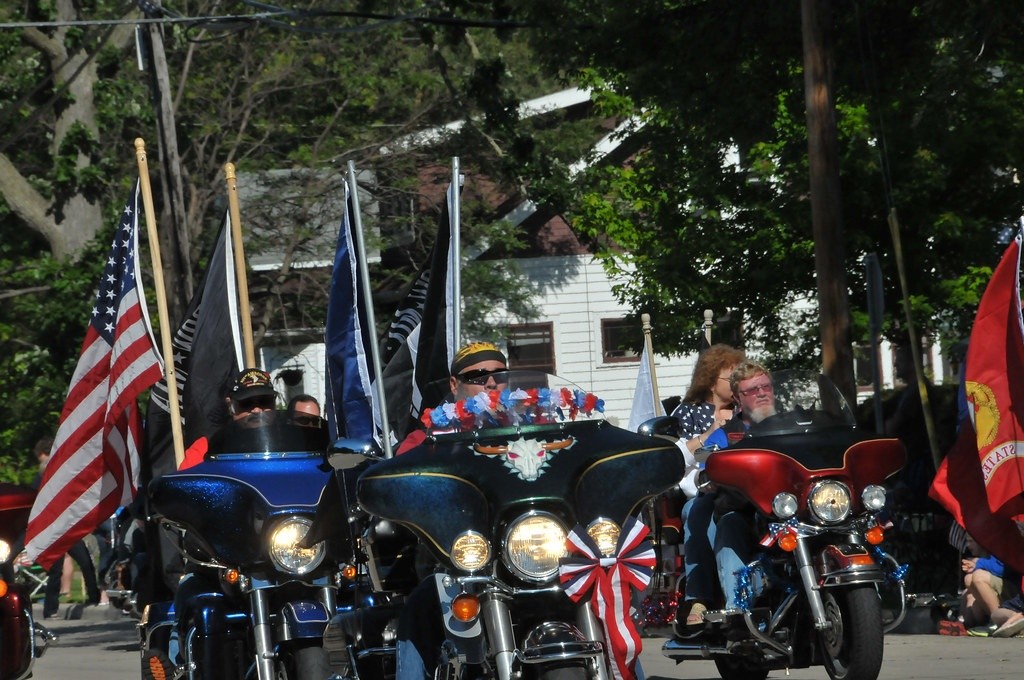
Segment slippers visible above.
[992,618,1024,638]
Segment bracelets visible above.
[698,436,704,447]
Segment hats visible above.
[227,368,279,401]
[451,341,506,378]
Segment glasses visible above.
[288,416,319,426]
[232,397,275,411]
[737,383,772,396]
[456,368,509,385]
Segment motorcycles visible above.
[328,371,686,680]
[662,369,907,680]
[0,481,58,680]
[98,410,371,680]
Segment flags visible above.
[323,178,384,453]
[24,174,166,571]
[926,233,1024,596]
[143,209,243,483]
[628,336,668,433]
[371,173,461,449]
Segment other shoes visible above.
[686,603,707,625]
[141,648,175,680]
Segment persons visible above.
[397,341,557,680]
[929,532,1024,638]
[284,394,320,428]
[141,368,279,680]
[669,343,787,626]
[852,340,936,515]
[27,438,101,622]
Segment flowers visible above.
[422,395,606,429]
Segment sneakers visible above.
[726,615,750,641]
